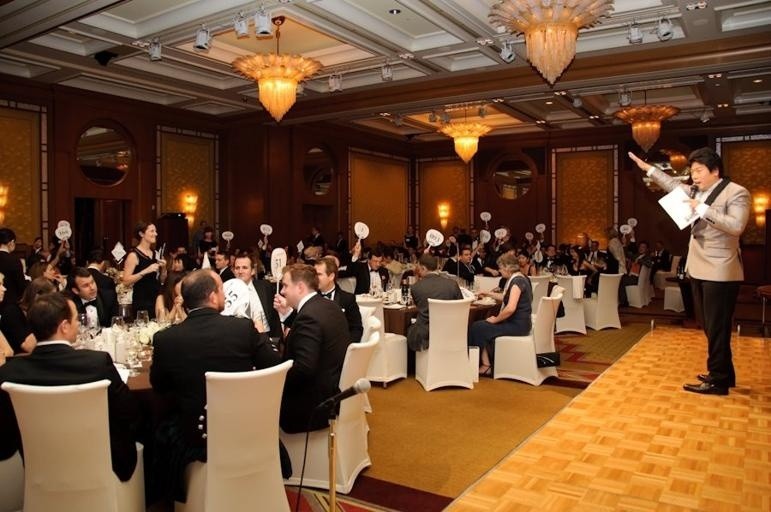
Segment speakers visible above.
[158,218,189,256]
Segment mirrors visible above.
[487,151,539,204]
[302,145,335,197]
[72,118,137,190]
[636,151,677,201]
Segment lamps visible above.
[193,23,213,50]
[612,90,682,151]
[150,36,163,61]
[573,96,582,108]
[656,16,674,41]
[699,111,712,126]
[429,105,492,163]
[628,21,644,45]
[380,59,394,81]
[396,115,402,127]
[486,0,614,86]
[233,3,324,124]
[328,70,342,92]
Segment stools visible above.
[751,283,771,328]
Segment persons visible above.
[627,148,751,395]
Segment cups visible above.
[455,275,482,300]
[252,311,263,329]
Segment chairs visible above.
[1,297,407,512]
[584,273,622,332]
[415,275,586,392]
[626,256,684,315]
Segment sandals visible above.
[478,363,493,378]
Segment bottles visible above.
[676,267,680,278]
[679,264,684,281]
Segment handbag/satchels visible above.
[534,351,562,369]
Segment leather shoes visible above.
[695,369,737,387]
[683,383,730,396]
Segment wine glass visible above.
[74,305,183,378]
[370,253,421,307]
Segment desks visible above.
[380,294,502,336]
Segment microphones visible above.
[690,185,698,199]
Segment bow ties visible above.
[84,299,98,308]
[370,268,378,273]
[321,292,332,299]
